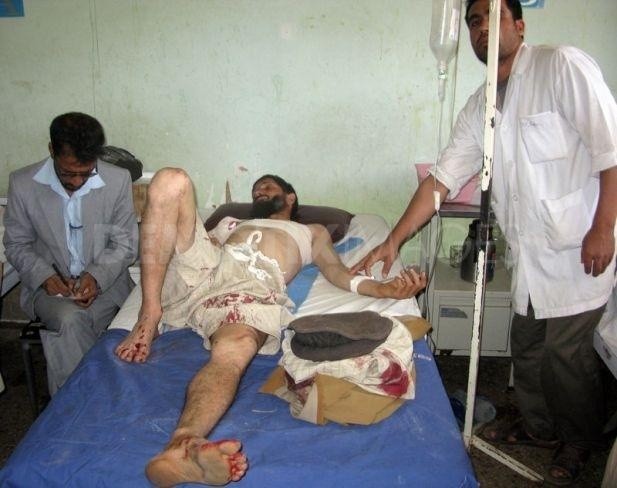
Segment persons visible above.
[115,167,427,488]
[349,0,617,486]
[3,113,139,398]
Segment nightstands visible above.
[420,189,515,393]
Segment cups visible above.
[450,244,462,266]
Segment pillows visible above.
[205,200,357,248]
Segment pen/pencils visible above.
[52,263,75,296]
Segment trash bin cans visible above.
[1,323,48,408]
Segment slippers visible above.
[545,441,588,485]
[482,417,562,446]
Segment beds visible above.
[1,212,479,488]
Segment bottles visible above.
[462,219,506,283]
[430,0,462,76]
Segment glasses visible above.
[54,155,99,177]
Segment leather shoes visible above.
[34,391,52,417]
